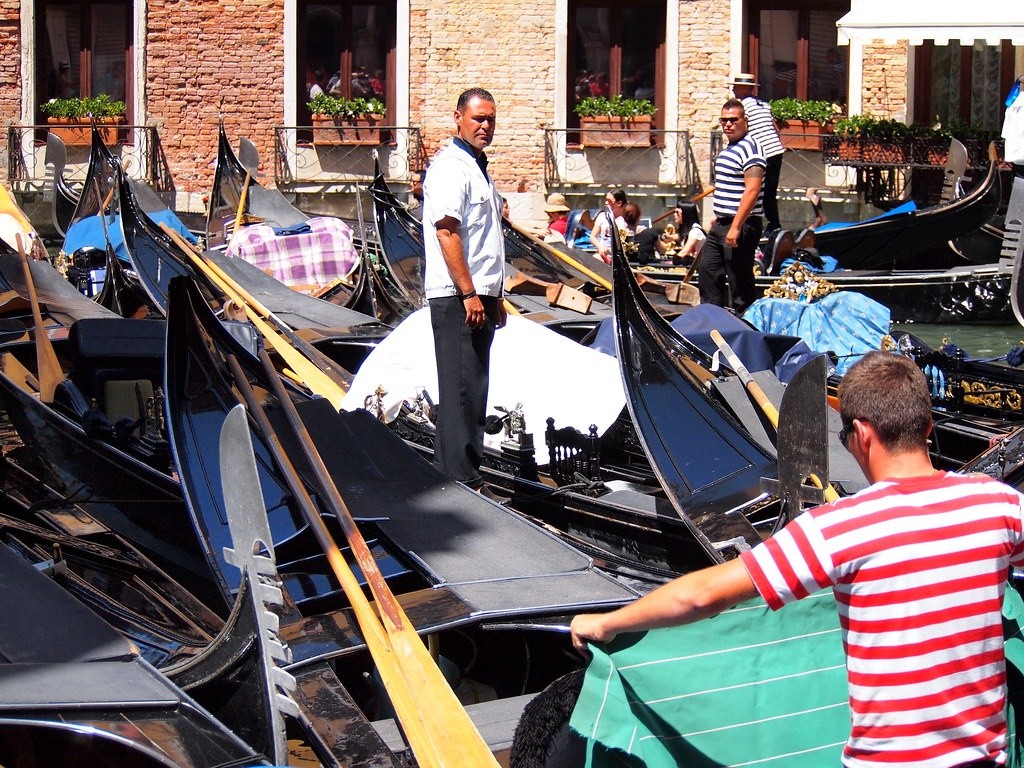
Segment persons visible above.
[398,170,426,221]
[422,89,512,505]
[38,57,124,102]
[306,66,385,101]
[503,188,707,263]
[574,65,609,101]
[698,99,767,315]
[569,353,1024,767]
[726,73,787,237]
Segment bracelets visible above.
[462,290,476,300]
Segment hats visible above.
[542,193,571,213]
[726,74,761,86]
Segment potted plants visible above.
[303,92,387,147]
[36,98,125,147]
[831,116,954,165]
[763,99,837,154]
[573,97,655,149]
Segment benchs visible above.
[565,208,608,249]
[764,226,817,276]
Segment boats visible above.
[0,114,1024,768]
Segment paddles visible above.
[710,329,841,506]
[96,159,131,217]
[159,220,355,413]
[15,232,66,403]
[224,168,251,258]
[500,216,613,293]
[190,276,503,768]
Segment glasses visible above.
[673,209,682,215]
[719,116,743,123]
[837,417,868,452]
[410,179,422,185]
[604,198,617,204]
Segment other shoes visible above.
[480,485,513,506]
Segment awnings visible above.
[835,0,1024,46]
